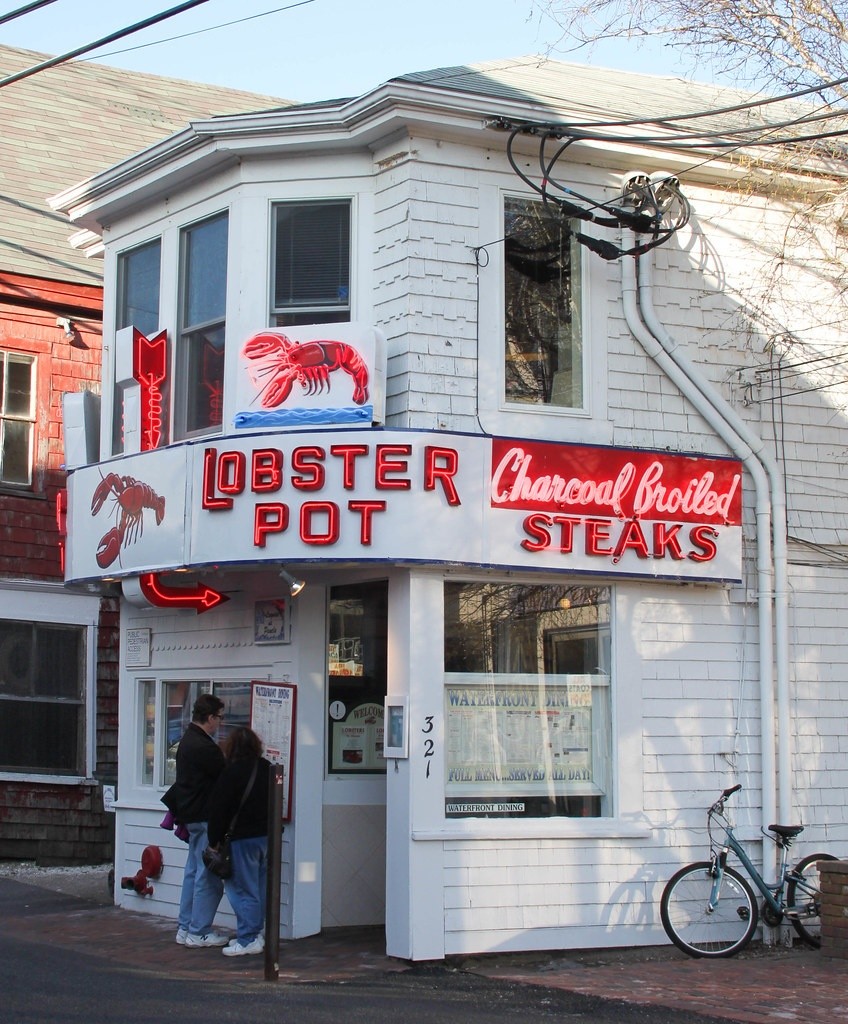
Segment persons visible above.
[159,694,228,947]
[204,726,273,956]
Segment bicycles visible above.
[659,783,841,959]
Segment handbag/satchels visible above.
[160,780,204,822]
[202,833,233,877]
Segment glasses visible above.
[217,715,224,721]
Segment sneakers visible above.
[176,929,187,944]
[186,933,229,947]
[221,934,265,955]
